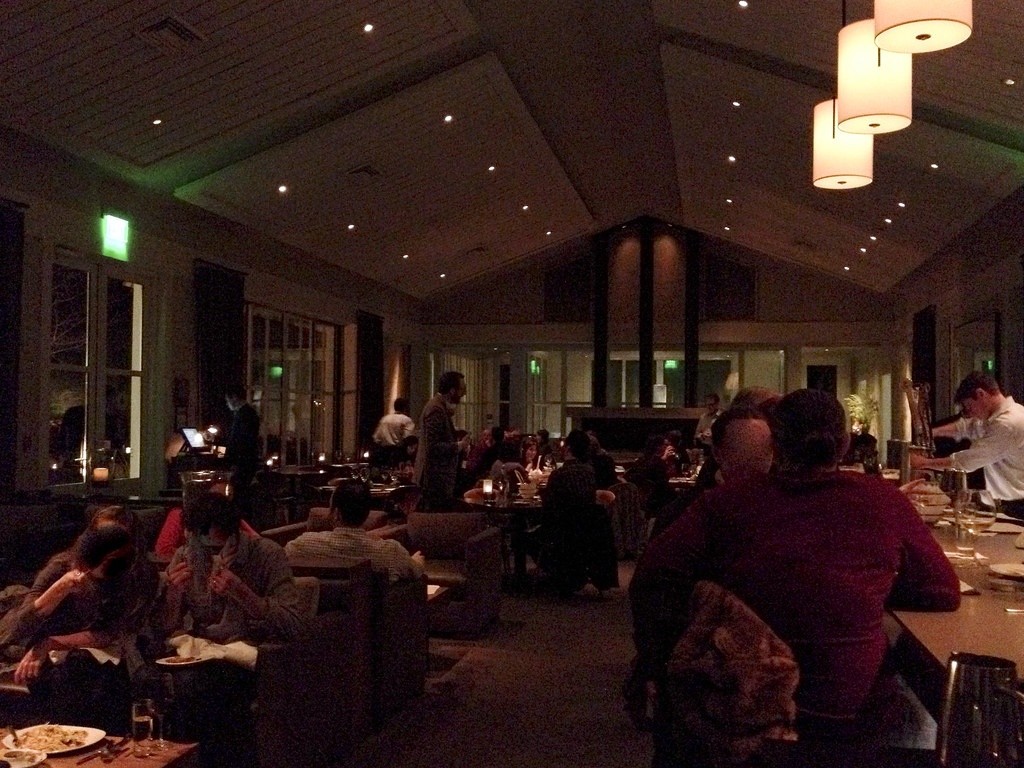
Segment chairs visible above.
[658,581,937,768]
[602,484,658,562]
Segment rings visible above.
[213,581,216,586]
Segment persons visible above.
[0,474,425,768]
[372,372,615,604]
[198,384,260,517]
[624,388,961,737]
[911,370,1024,521]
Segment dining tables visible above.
[838,465,900,487]
[0,727,199,768]
[887,518,1024,691]
[668,464,703,490]
[464,489,548,587]
[316,480,410,511]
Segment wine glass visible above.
[941,468,967,539]
[144,673,175,755]
[955,489,996,561]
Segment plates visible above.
[990,563,1024,576]
[0,749,47,768]
[156,656,196,664]
[1,725,106,753]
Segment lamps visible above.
[812,0,975,190]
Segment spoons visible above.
[103,748,129,764]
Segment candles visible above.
[94,467,108,481]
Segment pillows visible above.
[293,575,321,619]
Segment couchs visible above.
[118,512,505,768]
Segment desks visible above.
[169,456,229,489]
[271,463,367,495]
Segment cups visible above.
[132,699,153,756]
[515,469,535,501]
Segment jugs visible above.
[936,651,1024,768]
